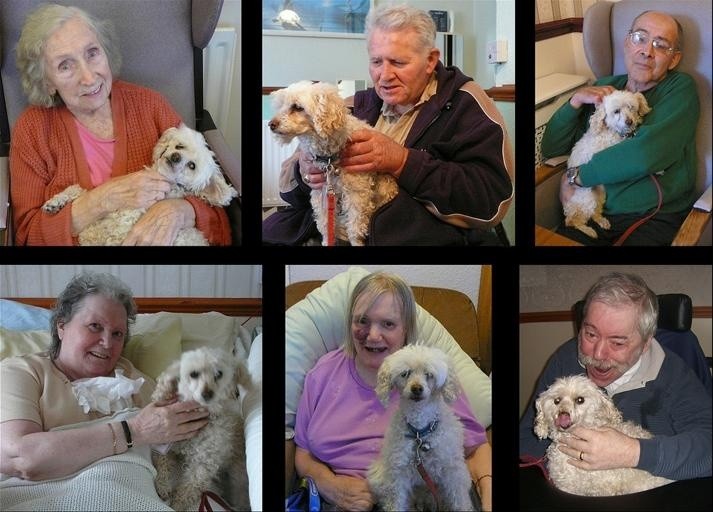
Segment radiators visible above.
[197,26,238,142]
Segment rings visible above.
[580,451,584,461]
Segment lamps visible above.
[273,8,301,26]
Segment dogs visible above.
[40,121,239,246]
[150,345,250,511]
[562,87,652,239]
[268,79,399,246]
[365,339,475,512]
[533,372,678,497]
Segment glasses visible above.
[628,31,676,56]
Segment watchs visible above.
[566,166,582,188]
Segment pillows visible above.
[283,263,494,440]
[0,319,187,382]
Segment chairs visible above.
[282,280,482,509]
[570,288,712,405]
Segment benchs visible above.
[534,72,594,172]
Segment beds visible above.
[0,290,238,512]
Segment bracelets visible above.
[108,423,117,455]
[475,474,492,485]
[120,420,133,448]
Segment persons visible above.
[294,269,492,512]
[518,271,713,481]
[261,2,516,245]
[0,270,209,482]
